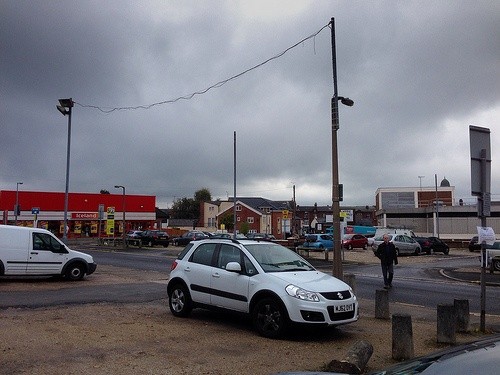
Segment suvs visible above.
[141,230,170,248]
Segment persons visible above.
[375,234,398,290]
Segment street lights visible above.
[331,95,354,279]
[15,182,23,225]
[56,97,75,243]
[114,185,127,249]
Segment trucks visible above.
[0,225,97,281]
[486,240,500,271]
[367,226,419,248]
[325,223,376,238]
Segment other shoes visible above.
[383,284,393,288]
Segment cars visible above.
[126,230,143,246]
[368,334,500,375]
[203,230,276,241]
[340,234,368,250]
[172,231,206,247]
[371,233,422,256]
[166,238,359,339]
[303,233,333,251]
[468,235,481,251]
[414,236,449,255]
